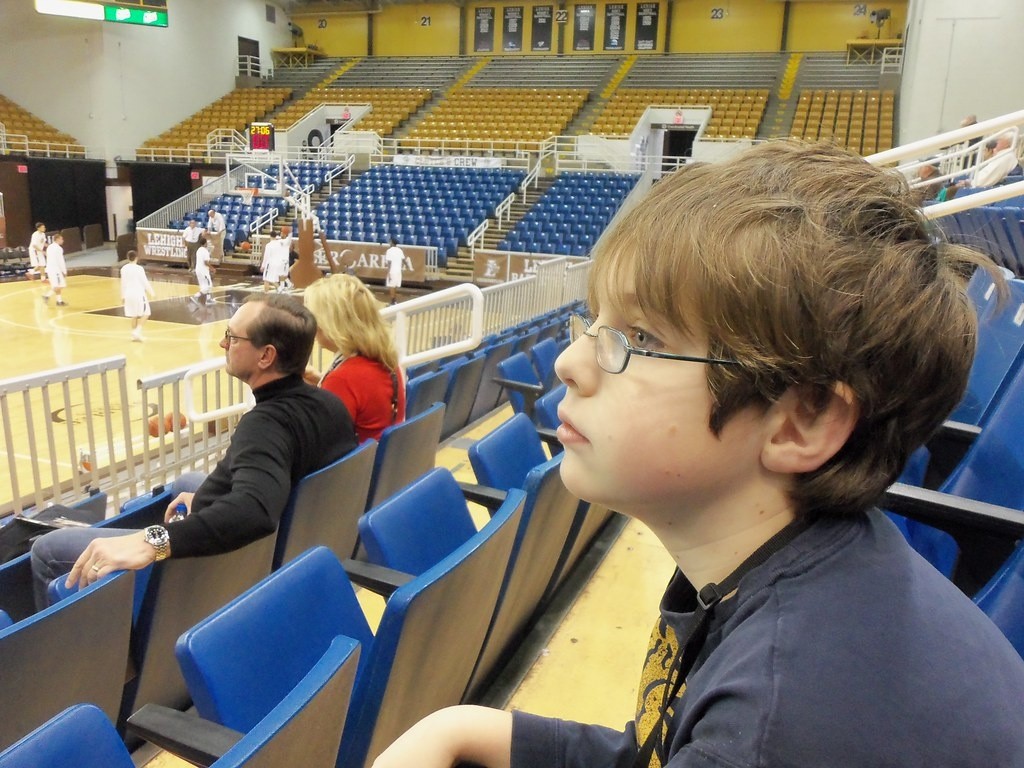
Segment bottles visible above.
[169,503,187,523]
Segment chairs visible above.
[0,50,1024,768]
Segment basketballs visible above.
[281,225,290,233]
[149,412,186,438]
[242,241,250,251]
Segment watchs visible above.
[144,525,169,562]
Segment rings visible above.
[91,566,100,572]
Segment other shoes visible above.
[206,299,216,306]
[42,280,49,283]
[57,302,67,305]
[43,295,49,304]
[25,272,34,281]
[190,296,199,306]
[132,331,144,341]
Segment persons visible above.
[43,234,68,305]
[207,210,226,261]
[940,114,982,170]
[385,240,409,305]
[260,229,299,294]
[303,273,407,445]
[121,251,151,342]
[372,140,1024,768]
[31,294,359,611]
[182,220,206,272]
[25,223,49,283]
[195,238,217,304]
[915,156,943,201]
[946,131,1021,200]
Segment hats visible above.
[391,235,399,244]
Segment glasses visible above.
[225,332,255,345]
[569,313,797,375]
